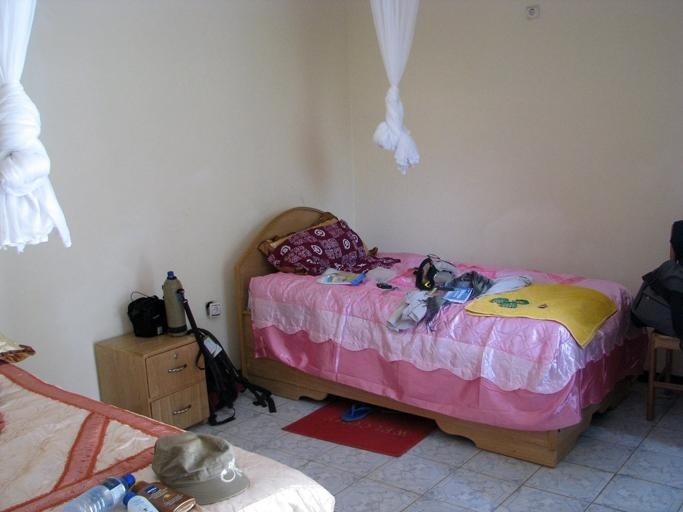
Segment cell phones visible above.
[377,283,392,289]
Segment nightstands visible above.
[94,329,210,431]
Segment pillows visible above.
[258,212,379,274]
[0,330,35,365]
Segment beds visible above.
[234,207,649,467]
[0,364,336,512]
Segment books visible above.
[440,288,472,304]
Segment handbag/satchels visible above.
[127,291,167,338]
[415,253,457,291]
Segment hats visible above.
[151,431,248,505]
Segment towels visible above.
[464,283,619,348]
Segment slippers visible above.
[341,402,372,422]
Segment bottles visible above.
[62,473,136,512]
[161,271,187,337]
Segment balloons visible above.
[315,266,366,286]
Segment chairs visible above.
[647,219,683,420]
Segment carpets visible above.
[282,398,437,458]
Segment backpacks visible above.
[629,259,682,338]
[188,328,276,426]
